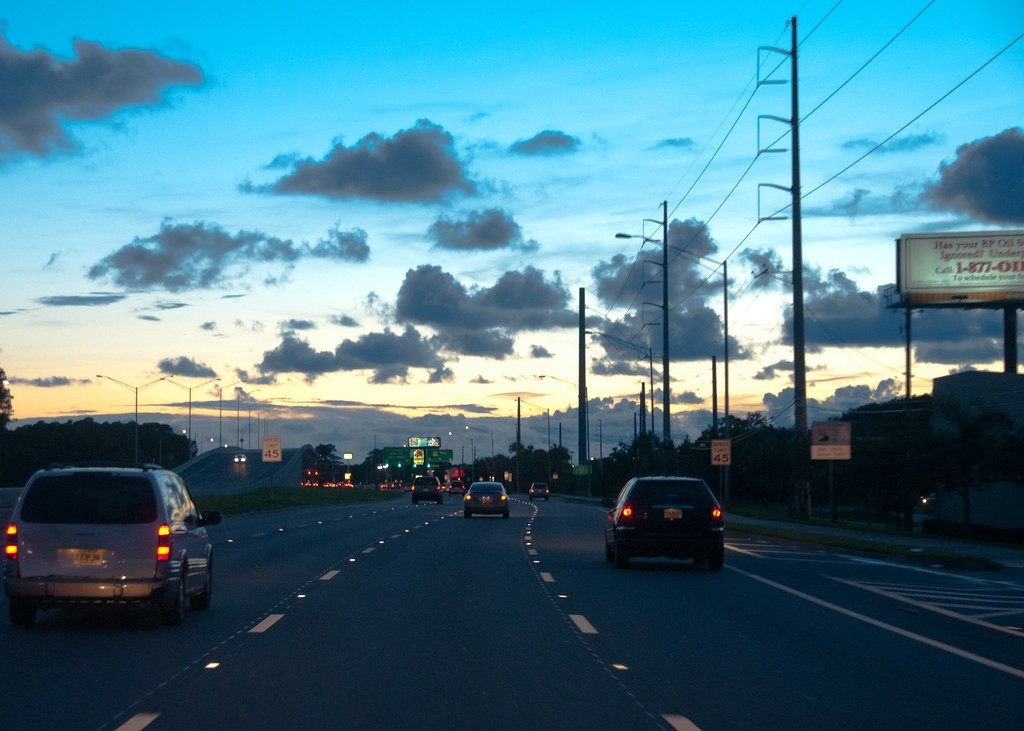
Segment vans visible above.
[3,463,222,627]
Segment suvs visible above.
[600,474,726,571]
[443,480,465,497]
[528,482,550,501]
[411,476,443,504]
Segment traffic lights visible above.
[398,464,401,467]
[413,464,416,467]
[427,463,431,468]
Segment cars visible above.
[233,453,248,463]
[463,482,510,518]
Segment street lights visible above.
[465,426,494,475]
[237,389,280,450]
[614,232,730,440]
[159,377,221,459]
[208,379,245,446]
[515,399,550,452]
[96,373,174,468]
[448,432,473,482]
[539,376,590,461]
[585,331,654,435]
